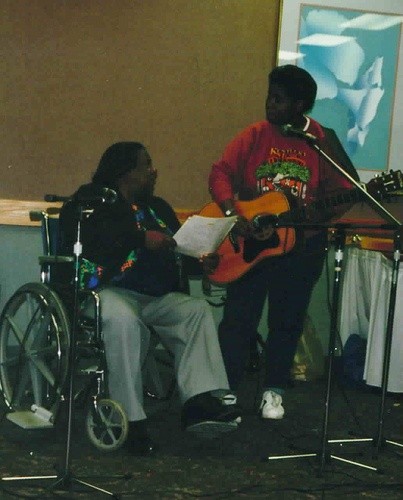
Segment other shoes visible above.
[122,420,154,458]
[182,392,241,426]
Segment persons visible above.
[209,64,360,422]
[52,141,243,454]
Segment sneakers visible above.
[259,390,285,420]
[221,394,241,424]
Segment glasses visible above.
[129,165,157,181]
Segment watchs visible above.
[226,207,237,215]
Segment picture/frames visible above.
[277,0,403,183]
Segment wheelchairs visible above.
[1,186,240,455]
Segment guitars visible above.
[197,168,403,287]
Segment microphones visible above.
[279,123,318,143]
[44,187,118,205]
